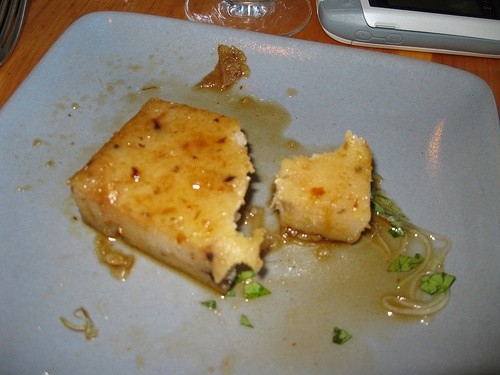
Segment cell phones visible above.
[314,0,500,59]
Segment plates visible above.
[0,10,500,375]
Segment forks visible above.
[0,0,26,67]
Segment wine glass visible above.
[183,0,311,39]
